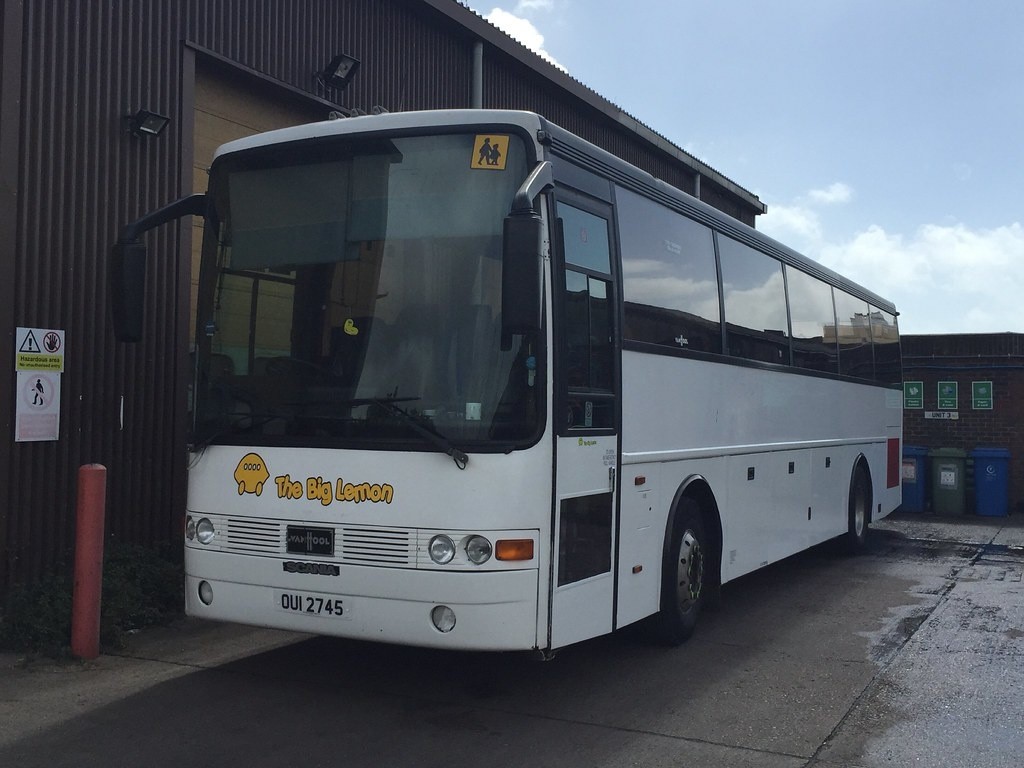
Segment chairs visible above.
[338,303,494,356]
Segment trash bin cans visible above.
[968,447,1012,516]
[930,448,968,513]
[896,445,929,511]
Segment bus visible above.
[108,108,903,660]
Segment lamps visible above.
[314,53,359,89]
[128,108,170,138]
